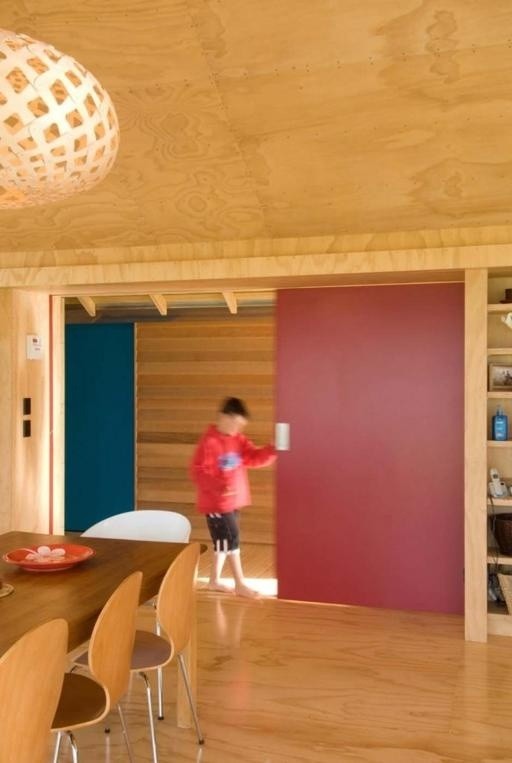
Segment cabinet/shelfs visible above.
[465,268,512,646]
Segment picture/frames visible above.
[489,363,511,391]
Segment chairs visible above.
[78,541,205,747]
[61,571,135,763]
[0,618,68,763]
[78,509,191,721]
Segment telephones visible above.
[487,466,508,497]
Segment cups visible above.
[274,422,291,451]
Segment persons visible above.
[188,394,281,600]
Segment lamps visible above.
[0,29,120,210]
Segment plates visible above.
[3,545,97,572]
